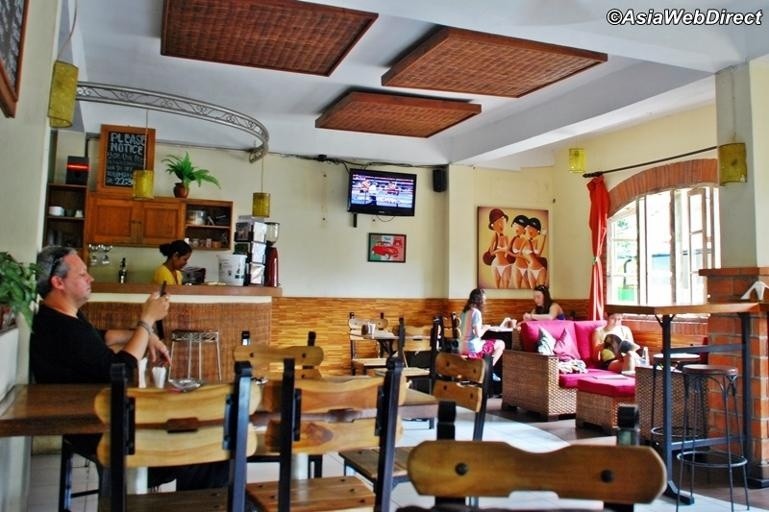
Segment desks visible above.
[604,301,761,505]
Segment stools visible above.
[165,330,223,384]
[676,364,750,511]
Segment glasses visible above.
[537,285,544,290]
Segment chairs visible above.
[347,312,389,376]
[337,346,495,512]
[407,439,668,511]
[439,312,461,353]
[232,330,325,379]
[93,361,262,512]
[649,352,711,485]
[246,357,408,511]
[369,317,441,429]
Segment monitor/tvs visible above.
[348,169,417,218]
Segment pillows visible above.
[532,326,558,356]
[552,327,581,362]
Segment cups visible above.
[151,366,166,389]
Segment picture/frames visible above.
[367,232,407,263]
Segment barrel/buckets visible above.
[216,253,247,286]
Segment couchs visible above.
[501,319,623,422]
[635,336,708,453]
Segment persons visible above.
[458,288,504,382]
[518,219,547,287]
[155,241,192,286]
[31,247,228,491]
[594,309,641,376]
[522,282,565,326]
[359,181,399,203]
[482,208,513,289]
[505,215,529,290]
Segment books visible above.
[602,333,639,353]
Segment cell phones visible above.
[158,282,167,299]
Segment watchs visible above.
[138,321,155,336]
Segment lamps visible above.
[46,57,80,130]
[718,142,747,187]
[567,142,587,173]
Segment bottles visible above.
[119,257,127,284]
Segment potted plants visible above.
[0,251,47,334]
[160,149,223,198]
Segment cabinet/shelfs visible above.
[42,183,89,268]
[84,192,234,251]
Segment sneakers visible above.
[492,373,501,381]
